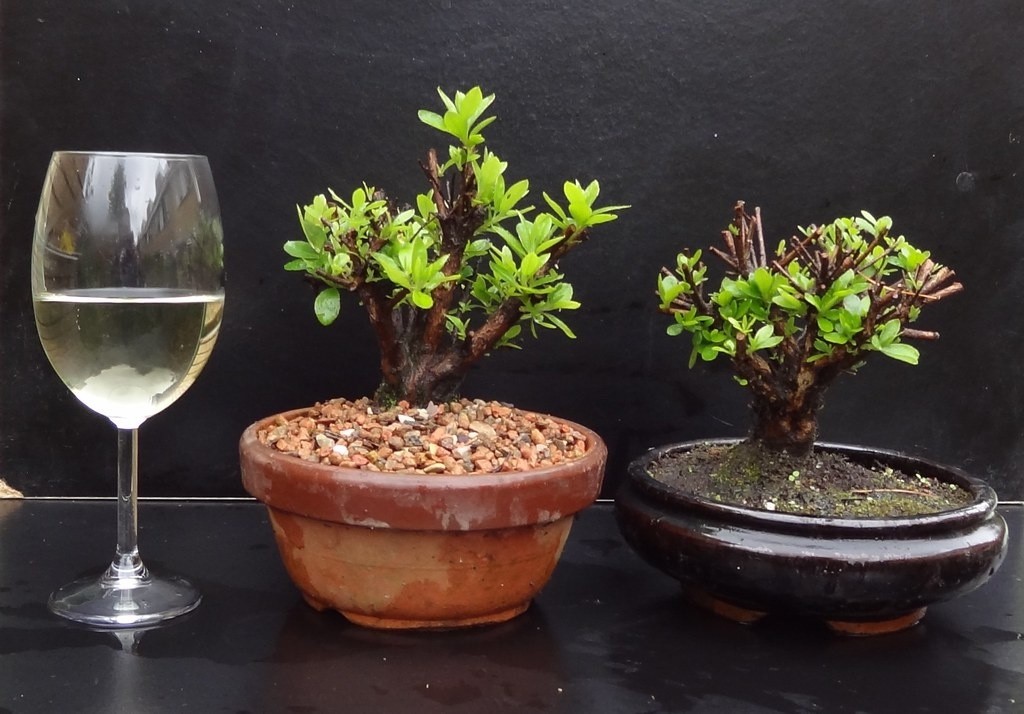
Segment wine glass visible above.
[31,150,226,628]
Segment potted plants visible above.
[238,82,629,629]
[613,198,1008,635]
